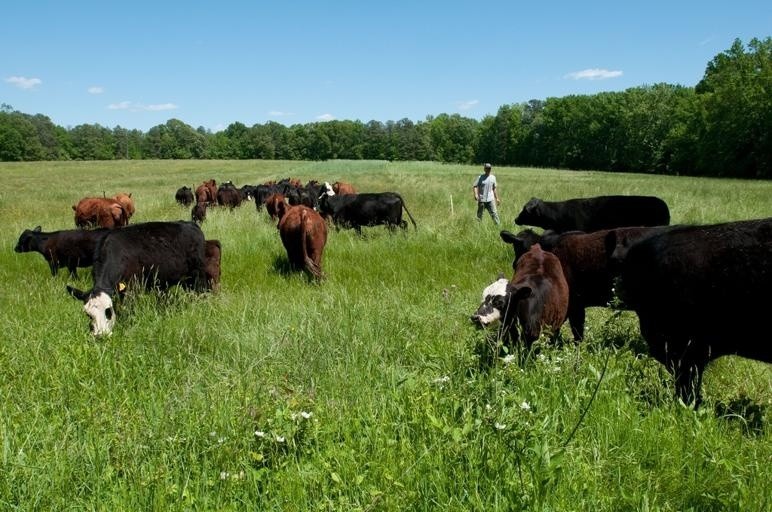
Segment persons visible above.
[473,163,500,226]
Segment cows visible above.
[15,191,224,341]
[472,193,679,366]
[316,191,419,238]
[175,176,357,221]
[606,217,772,410]
[276,200,328,286]
